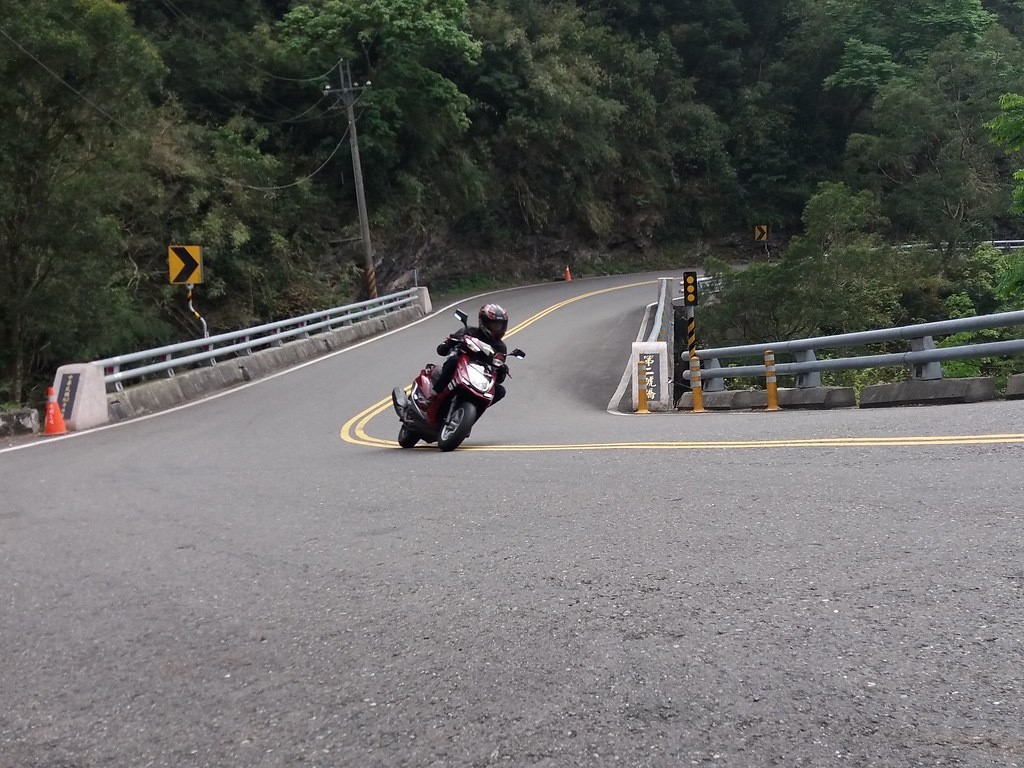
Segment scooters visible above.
[391,309,526,452]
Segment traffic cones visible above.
[39,386,69,437]
[564,265,573,283]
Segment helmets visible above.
[478,304,508,341]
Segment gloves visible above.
[447,333,458,347]
[499,364,509,374]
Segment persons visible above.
[420,304,510,408]
